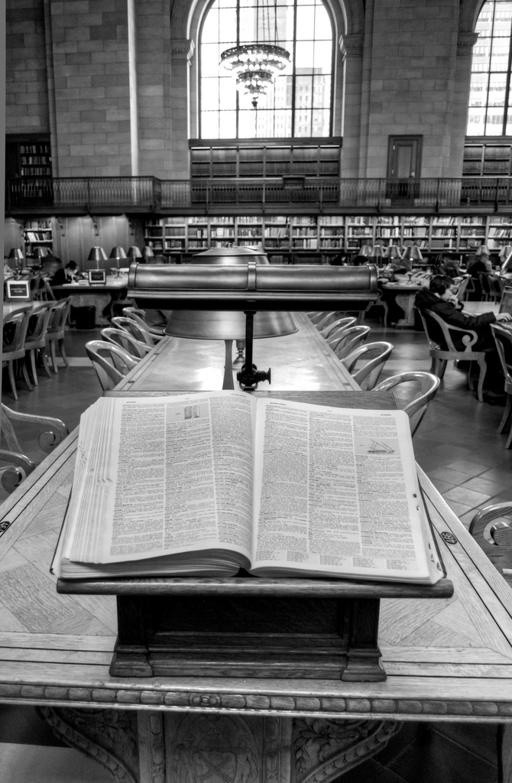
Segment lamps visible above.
[231,247,269,372]
[87,245,154,280]
[219,0,292,109]
[358,245,423,284]
[161,246,298,390]
[475,245,512,272]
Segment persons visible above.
[414,277,511,393]
[49,261,80,301]
[332,249,512,324]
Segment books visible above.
[159,216,512,249]
[51,386,446,587]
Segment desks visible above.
[1,310,512,782]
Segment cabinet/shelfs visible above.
[145,214,512,251]
[24,229,55,267]
[5,132,51,210]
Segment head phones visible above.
[438,276,446,295]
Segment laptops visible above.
[498,292,512,321]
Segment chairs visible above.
[0,269,511,586]
